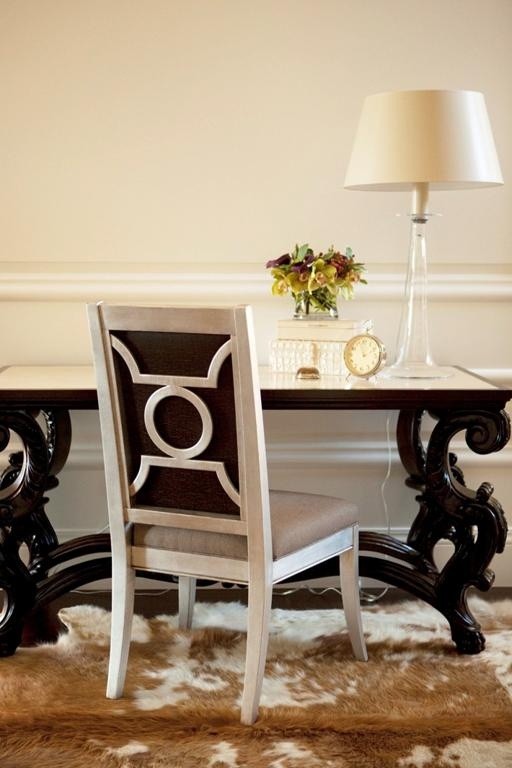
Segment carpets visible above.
[0,592,511,767]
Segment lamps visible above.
[343,88,505,379]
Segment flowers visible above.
[266,243,369,314]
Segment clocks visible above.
[342,321,388,380]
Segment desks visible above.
[0,364,512,658]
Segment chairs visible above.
[84,299,370,728]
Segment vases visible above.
[292,289,339,320]
